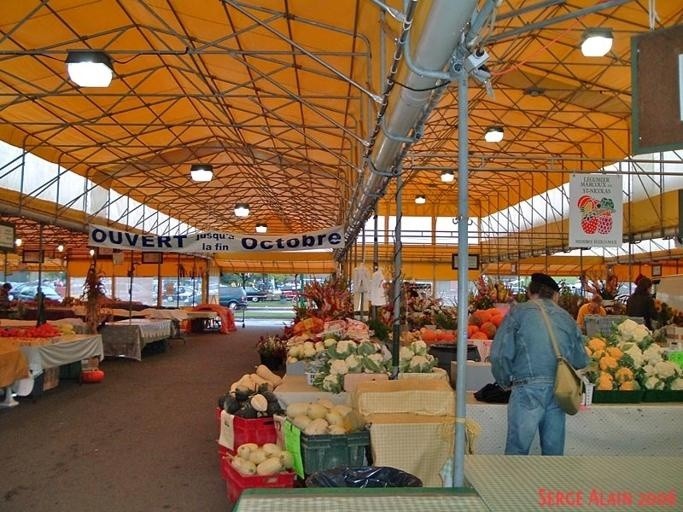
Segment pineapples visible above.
[597,215,613,234]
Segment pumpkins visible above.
[231,443,293,476]
[287,398,366,436]
[228,364,282,397]
[82,368,104,383]
[421,308,504,344]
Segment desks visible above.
[107,296,237,362]
[0,301,107,408]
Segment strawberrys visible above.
[581,216,598,234]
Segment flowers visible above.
[254,327,288,354]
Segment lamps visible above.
[440,170,454,182]
[187,164,214,183]
[578,27,615,58]
[484,126,503,143]
[61,48,114,91]
[234,203,250,218]
[254,222,267,234]
[413,195,426,204]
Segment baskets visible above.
[585,315,644,337]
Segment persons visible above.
[34,286,46,299]
[403,282,418,312]
[625,278,667,331]
[489,272,588,455]
[576,293,605,334]
[601,274,618,300]
[0,282,17,309]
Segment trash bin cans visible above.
[304,466,423,487]
[280,296,287,304]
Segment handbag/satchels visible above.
[554,357,585,415]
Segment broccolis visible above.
[584,318,683,390]
[288,339,434,391]
